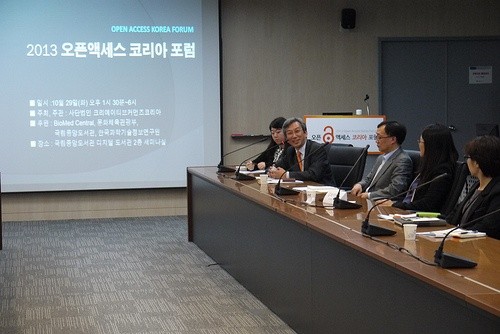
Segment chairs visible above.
[328,146,368,191]
[442,161,469,218]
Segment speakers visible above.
[341,9,356,29]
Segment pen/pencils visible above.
[244,161,256,165]
[461,230,478,234]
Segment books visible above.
[394,216,447,227]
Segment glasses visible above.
[375,132,392,140]
[416,139,425,146]
[462,154,472,161]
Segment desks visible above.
[186,166,500,334]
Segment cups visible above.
[403,224,417,240]
[260,175,268,184]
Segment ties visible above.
[296,151,302,172]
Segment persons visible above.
[441,134,500,242]
[375,121,463,211]
[265,116,338,187]
[351,119,414,201]
[245,116,288,172]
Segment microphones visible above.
[333,144,370,208]
[360,172,448,236]
[217,137,268,172]
[275,141,329,195]
[235,144,281,180]
